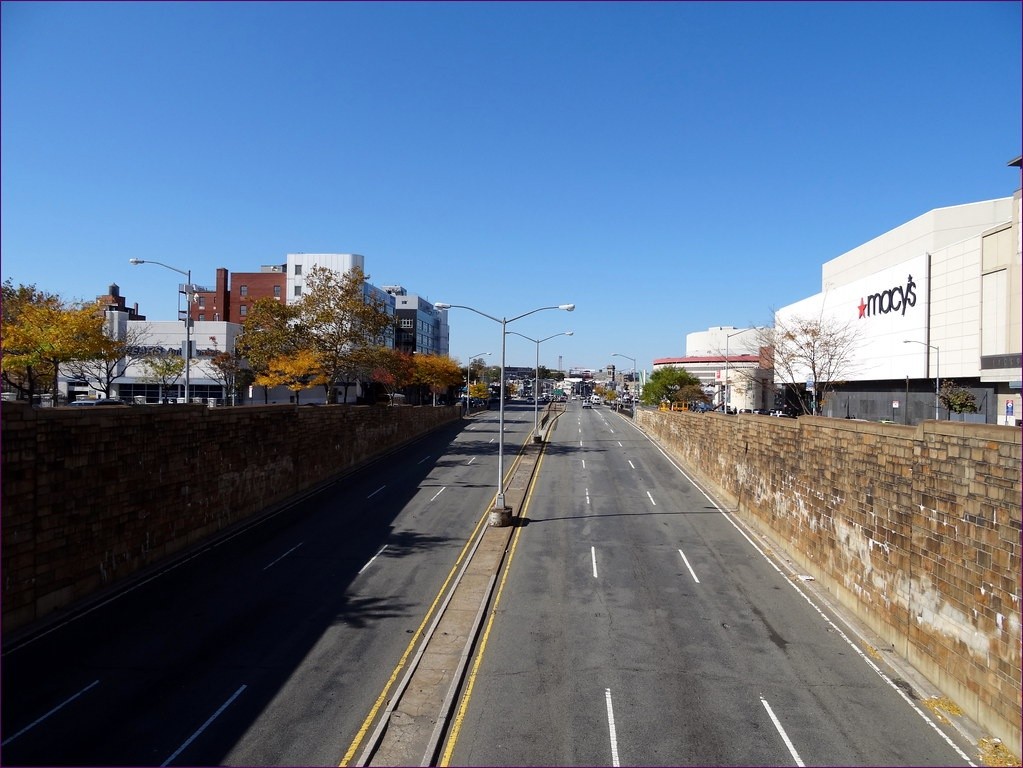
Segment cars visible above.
[527,398,534,404]
[538,393,567,403]
[66,399,126,406]
[572,395,600,409]
[739,407,790,418]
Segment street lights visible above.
[612,353,636,410]
[504,330,574,443]
[466,352,492,416]
[902,339,940,420]
[232,329,265,406]
[129,258,191,403]
[433,303,577,527]
[724,326,764,414]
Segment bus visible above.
[659,398,689,412]
